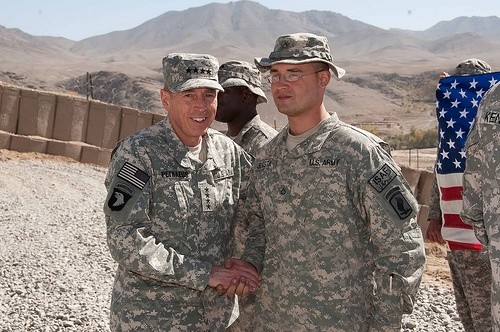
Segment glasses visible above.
[268,69,324,83]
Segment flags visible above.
[434,71,500,251]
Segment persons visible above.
[426,58,492,332]
[216,60,279,158]
[460,80,500,332]
[104,54,255,332]
[215,33,426,332]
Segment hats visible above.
[253,33,345,78]
[162,53,225,94]
[454,59,490,76]
[218,61,267,104]
